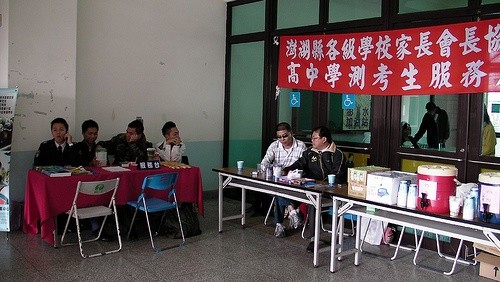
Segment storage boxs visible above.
[348,165,390,198]
[472,243,500,282]
[366,170,418,206]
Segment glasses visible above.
[276,131,289,139]
[311,137,321,140]
[126,130,136,136]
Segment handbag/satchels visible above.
[160,207,201,238]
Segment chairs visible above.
[265,196,477,275]
[60,177,123,258]
[126,172,186,252]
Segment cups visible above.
[147,148,155,161]
[327,174,335,186]
[462,197,474,221]
[449,196,460,217]
[236,161,244,170]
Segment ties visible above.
[58,146,62,157]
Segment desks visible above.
[324,184,500,282]
[22,163,204,246]
[212,167,347,267]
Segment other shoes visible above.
[274,223,285,238]
[306,241,315,253]
[288,214,303,229]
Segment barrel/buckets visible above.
[416,164,459,214]
[477,171,500,225]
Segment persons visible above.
[260,122,304,236]
[400,121,420,149]
[414,102,450,149]
[34,117,187,242]
[481,103,497,156]
[280,125,347,254]
